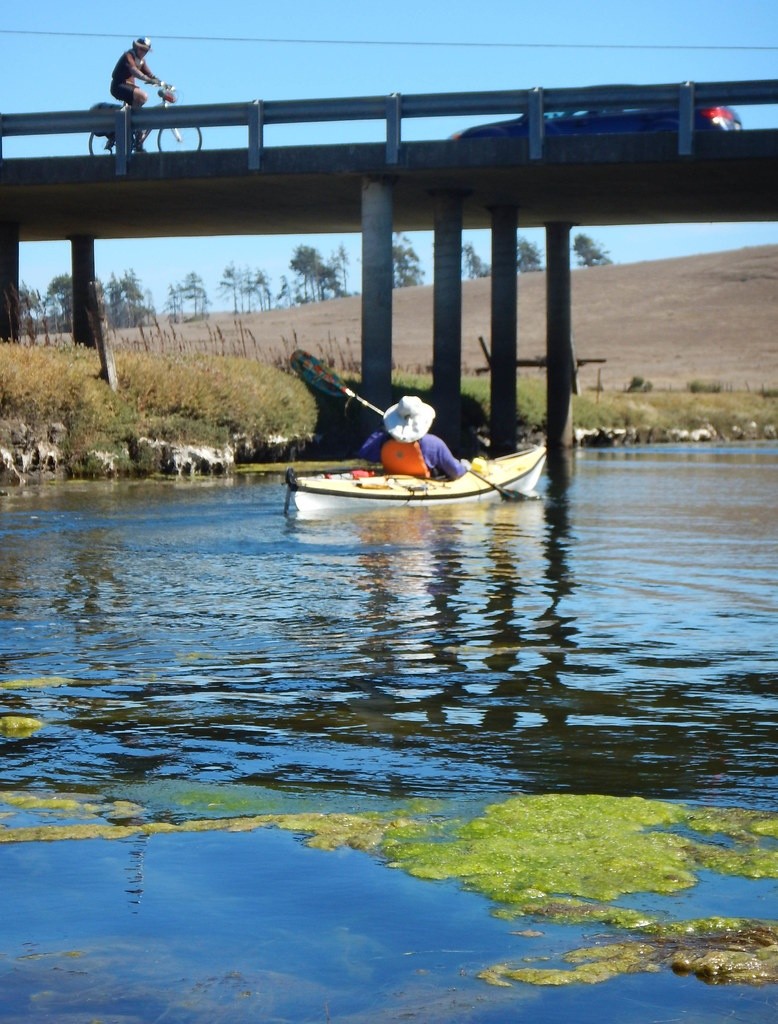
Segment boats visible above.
[293,446,548,501]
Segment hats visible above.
[133,37,151,51]
[383,396,436,442]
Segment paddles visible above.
[290,349,531,501]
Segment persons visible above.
[361,395,471,483]
[112,36,164,155]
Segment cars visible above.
[444,99,748,139]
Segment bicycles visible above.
[89,81,202,160]
